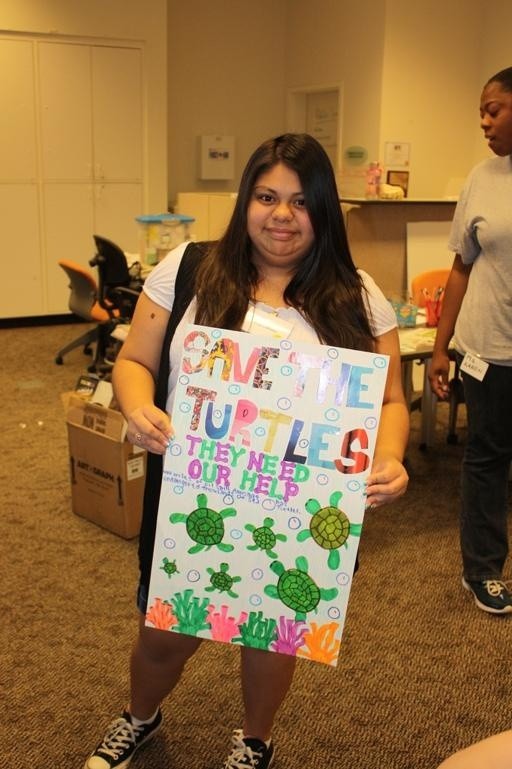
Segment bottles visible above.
[365,160,382,199]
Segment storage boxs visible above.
[60,390,147,539]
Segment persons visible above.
[82,132,412,768]
[428,68,512,615]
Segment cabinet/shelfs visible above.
[1,0,169,320]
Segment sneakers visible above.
[461,576,512,615]
[222,728,275,769]
[85,706,163,769]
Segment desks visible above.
[396,327,463,464]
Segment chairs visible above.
[408,269,451,316]
[56,235,145,382]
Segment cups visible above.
[424,300,441,328]
